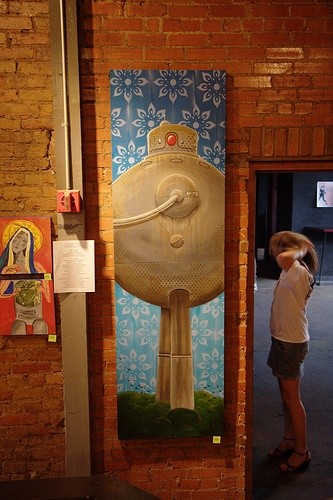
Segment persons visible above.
[319,185,327,202]
[267,231,319,472]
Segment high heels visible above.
[279,451,311,475]
[268,437,294,457]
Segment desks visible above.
[303,226,333,286]
